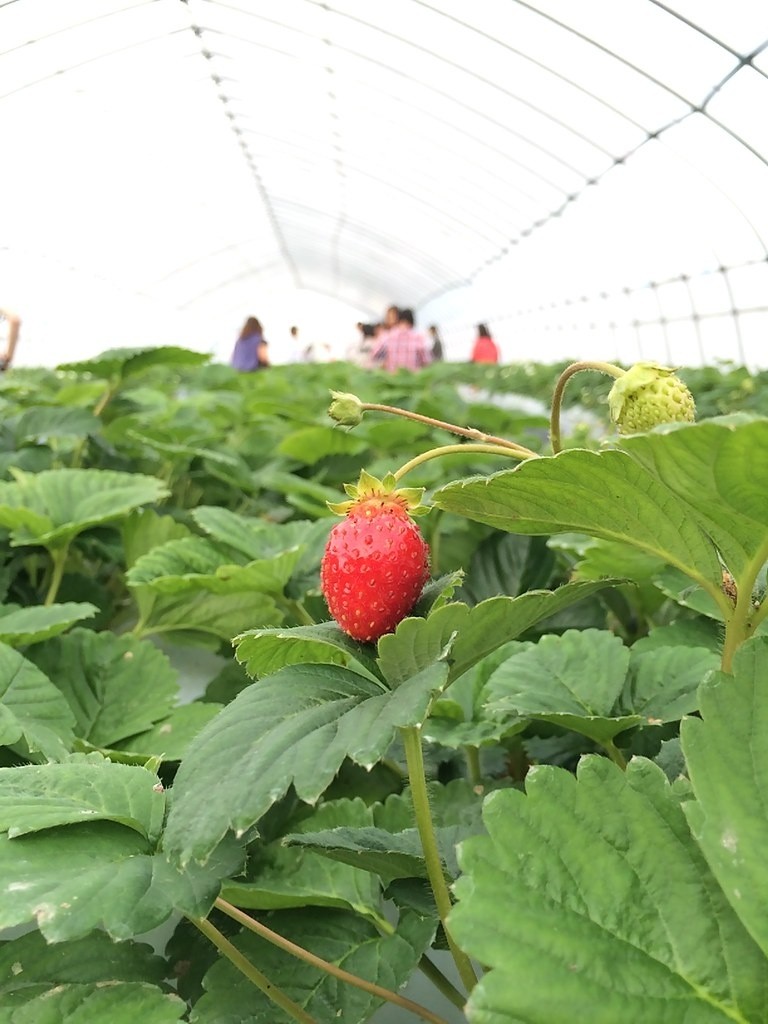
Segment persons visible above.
[290,306,498,371]
[231,317,271,372]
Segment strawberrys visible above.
[320,489,433,643]
[608,363,695,436]
